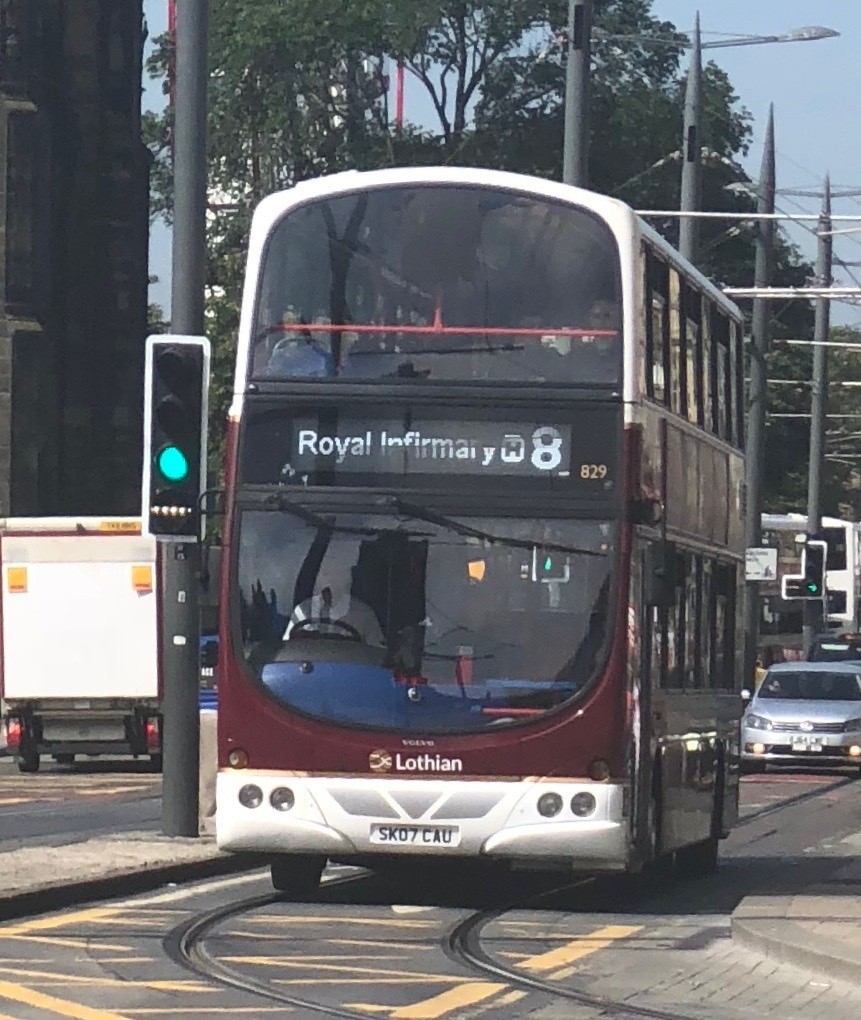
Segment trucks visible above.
[1,516,163,775]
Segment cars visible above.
[739,661,861,781]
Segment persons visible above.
[282,558,386,650]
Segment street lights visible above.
[676,25,841,269]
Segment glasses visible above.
[590,312,613,319]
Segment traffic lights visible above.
[142,334,211,544]
[783,541,828,599]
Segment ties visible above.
[319,587,334,635]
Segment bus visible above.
[761,512,861,637]
[194,165,752,912]
